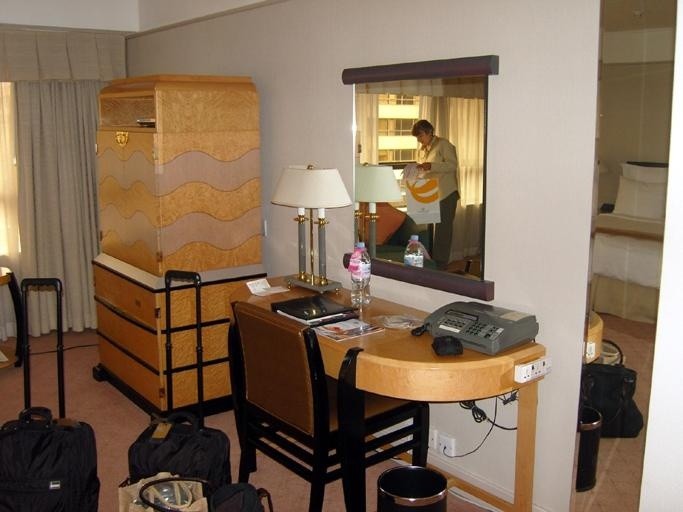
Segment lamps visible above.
[271,164,353,295]
[355,163,404,259]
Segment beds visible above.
[587,155,669,324]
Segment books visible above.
[270,293,358,324]
[311,318,385,342]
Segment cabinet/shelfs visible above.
[91,71,262,422]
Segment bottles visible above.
[404,235,424,267]
[348,242,372,306]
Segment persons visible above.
[412,120,460,269]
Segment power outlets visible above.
[428,429,436,449]
[540,355,552,376]
[439,432,455,458]
[514,364,532,383]
[527,359,544,381]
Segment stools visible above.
[0,266,24,369]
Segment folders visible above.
[271,296,360,327]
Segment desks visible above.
[226,272,548,512]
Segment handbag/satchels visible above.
[126,408,233,494]
[405,167,443,226]
[574,338,646,438]
[1,404,103,512]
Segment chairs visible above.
[227,301,431,512]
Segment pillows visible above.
[613,176,665,219]
